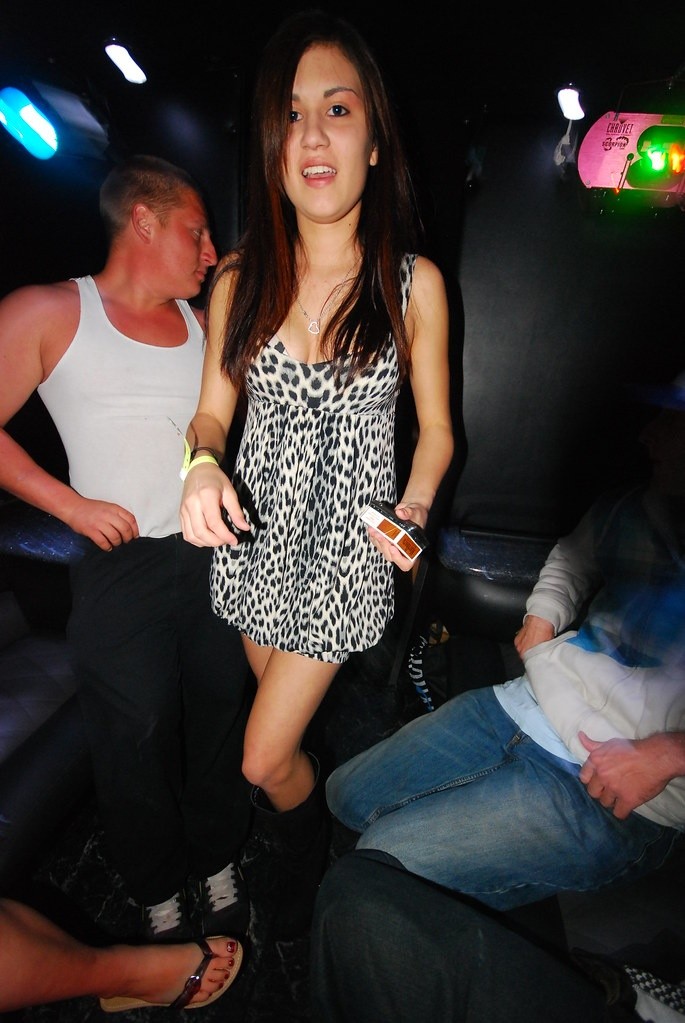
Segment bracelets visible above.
[191,447,218,462]
[168,416,219,482]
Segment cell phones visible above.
[371,499,430,550]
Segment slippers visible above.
[98,936,244,1013]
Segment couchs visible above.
[0,586,94,899]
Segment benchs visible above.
[555,867,684,990]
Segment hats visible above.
[626,368,685,412]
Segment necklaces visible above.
[296,258,361,335]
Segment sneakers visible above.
[140,863,253,945]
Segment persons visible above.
[0,157,252,944]
[179,14,456,938]
[325,372,685,914]
[0,900,244,1011]
[313,849,654,1023]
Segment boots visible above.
[250,751,328,976]
